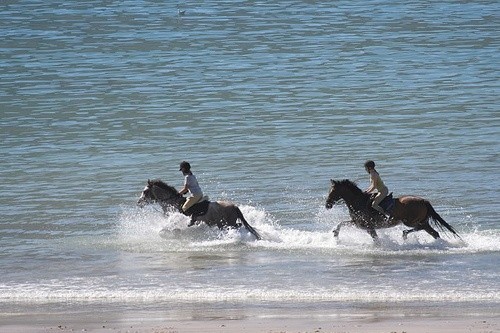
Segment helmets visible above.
[179,162,191,171]
[364,160,375,167]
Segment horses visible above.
[136,178,264,240]
[324,178,465,244]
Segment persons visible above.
[364,160,395,224]
[177,161,203,227]
